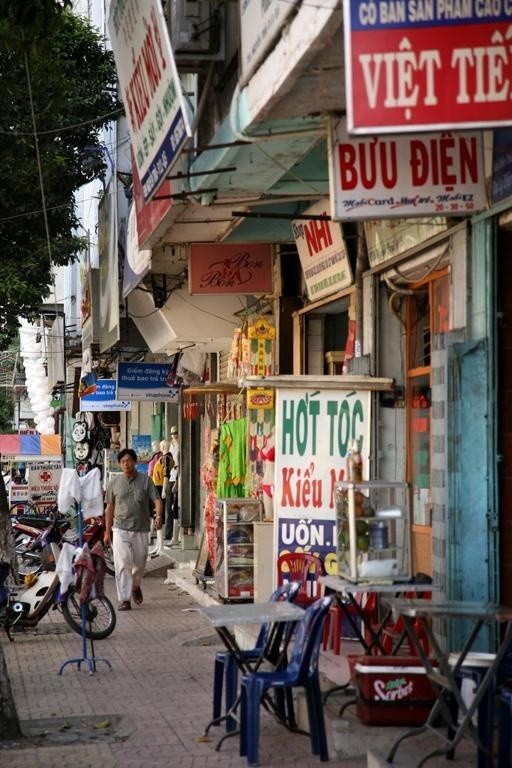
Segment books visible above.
[227,513,238,523]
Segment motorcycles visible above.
[0,491,118,642]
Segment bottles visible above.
[346,438,362,483]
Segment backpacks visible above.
[76,411,120,431]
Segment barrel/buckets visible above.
[447,651,499,728]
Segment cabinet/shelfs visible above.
[333,480,412,586]
[215,498,264,605]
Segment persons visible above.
[103,449,163,611]
[148,426,180,558]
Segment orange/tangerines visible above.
[338,491,375,552]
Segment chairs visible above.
[276,553,324,608]
[240,595,333,765]
[213,581,300,732]
[322,591,376,655]
[365,590,434,656]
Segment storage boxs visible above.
[346,654,440,725]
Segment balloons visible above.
[23,359,56,434]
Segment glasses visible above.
[169,432,179,435]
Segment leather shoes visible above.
[118,586,143,611]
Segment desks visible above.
[317,575,444,717]
[379,596,511,768]
[198,601,311,751]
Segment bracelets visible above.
[156,516,161,519]
